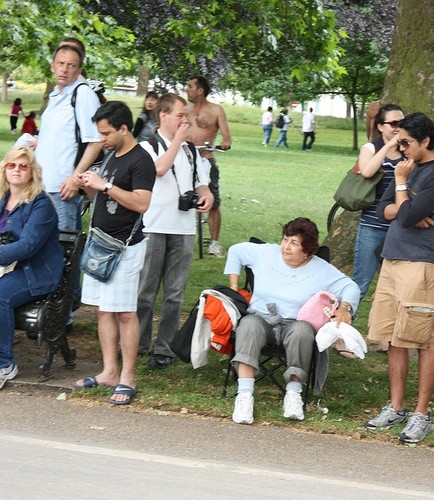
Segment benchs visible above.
[13,231,87,382]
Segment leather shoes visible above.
[148,354,174,369]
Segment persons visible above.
[33,37,104,329]
[14,130,40,152]
[71,100,156,404]
[0,145,64,388]
[261,106,276,147]
[302,107,315,150]
[365,111,434,443]
[275,109,293,149]
[9,98,26,134]
[137,93,214,370]
[132,91,159,144]
[21,112,39,134]
[184,76,232,255]
[222,217,360,425]
[350,104,408,319]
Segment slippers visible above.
[73,376,119,387]
[109,384,136,404]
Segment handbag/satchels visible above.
[74,144,106,195]
[79,227,126,283]
[170,294,206,363]
[333,163,384,212]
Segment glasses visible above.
[396,138,415,149]
[6,162,28,171]
[383,121,403,128]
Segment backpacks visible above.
[276,114,285,128]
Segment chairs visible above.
[178,238,331,411]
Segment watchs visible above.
[102,182,113,194]
[395,183,407,193]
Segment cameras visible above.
[178,190,205,211]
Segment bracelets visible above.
[340,305,352,311]
[230,285,238,287]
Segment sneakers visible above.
[399,412,432,442]
[366,405,408,430]
[283,390,304,421]
[0,363,18,389]
[232,394,254,424]
[209,240,221,254]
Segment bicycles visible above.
[194,141,230,260]
